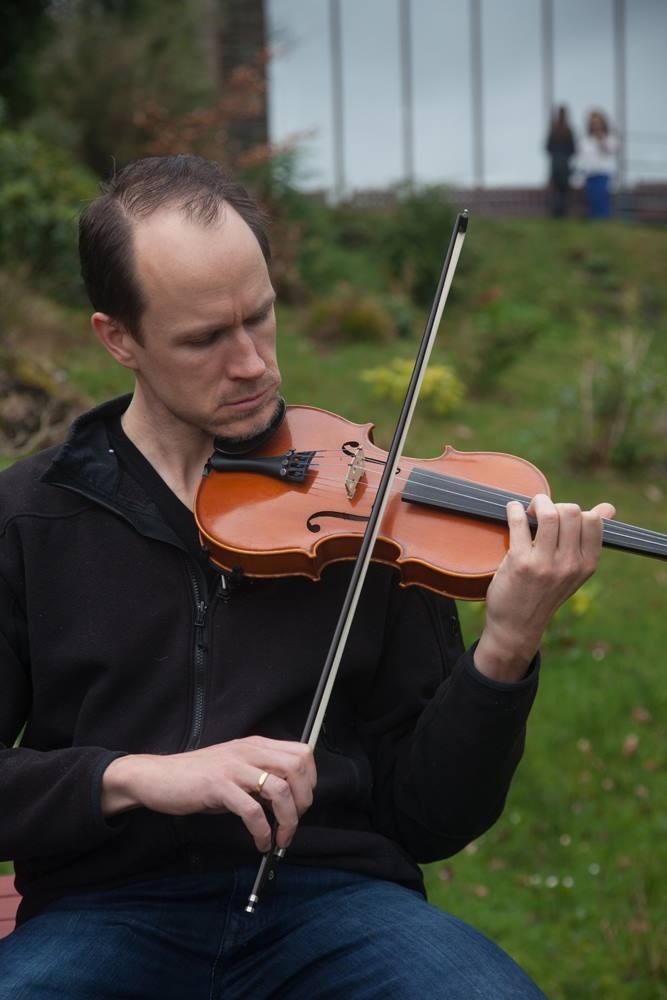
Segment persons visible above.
[577,112,618,221]
[545,107,575,219]
[0,158,615,1000]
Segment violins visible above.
[192,400,666,604]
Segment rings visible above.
[256,772,269,792]
